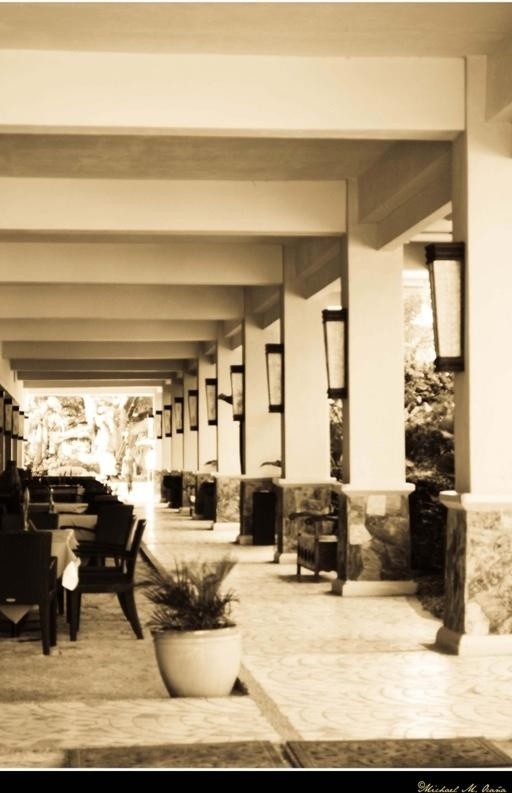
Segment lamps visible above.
[423,241,463,372]
[321,310,348,396]
[2,387,28,469]
[263,341,284,414]
[149,362,243,440]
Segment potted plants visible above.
[138,539,246,697]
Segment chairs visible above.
[287,509,339,582]
[185,484,196,515]
[162,473,182,511]
[0,469,147,658]
